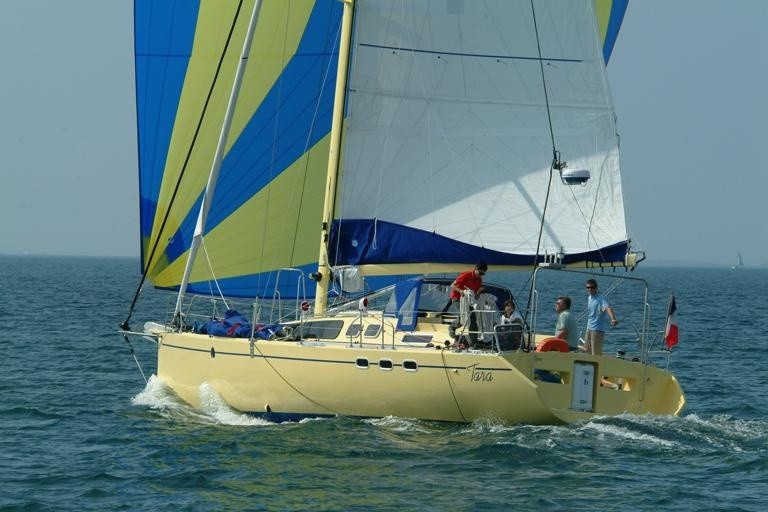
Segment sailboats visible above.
[115,1,688,438]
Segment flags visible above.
[663,293,678,349]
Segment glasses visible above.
[477,269,486,277]
[586,285,597,291]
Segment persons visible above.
[448,263,488,349]
[583,279,618,355]
[554,296,576,351]
[500,300,522,326]
